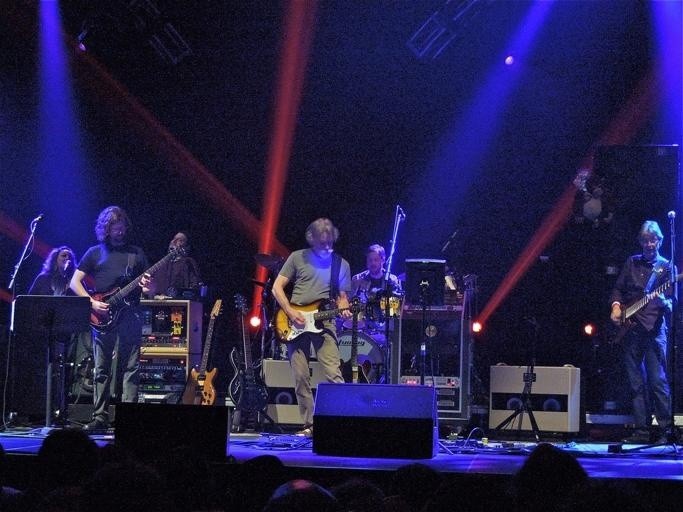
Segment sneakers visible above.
[295,427,313,437]
[83,419,108,434]
[621,433,675,445]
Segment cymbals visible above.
[255,252,287,271]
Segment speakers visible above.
[113,402,231,456]
[311,381,439,459]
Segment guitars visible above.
[274,298,376,344]
[180,300,224,406]
[229,294,268,412]
[89,245,187,330]
[606,274,682,348]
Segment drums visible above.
[333,330,391,384]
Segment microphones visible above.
[667,210,676,232]
[33,213,46,223]
[398,207,406,221]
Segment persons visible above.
[269,217,352,437]
[141,228,205,299]
[606,216,678,447]
[29,244,83,423]
[0,417,682,512]
[344,239,399,385]
[67,204,158,434]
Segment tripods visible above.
[0,222,47,432]
[487,324,544,442]
[620,232,683,454]
[26,335,87,430]
